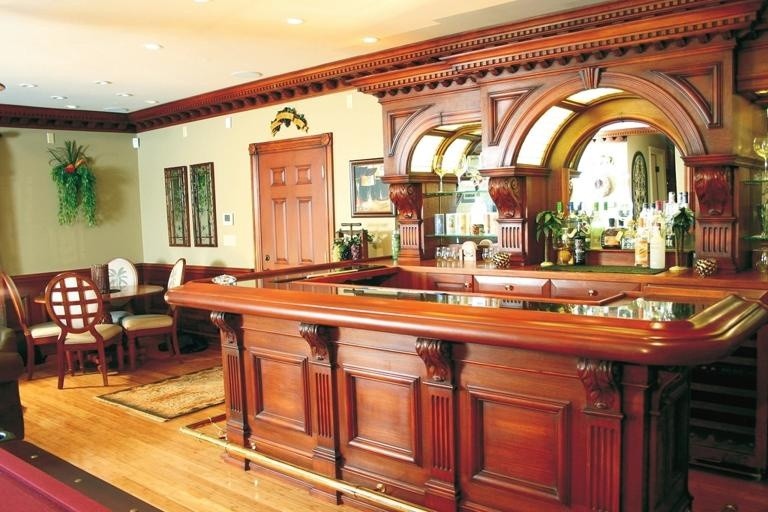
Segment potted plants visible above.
[43,139,106,231]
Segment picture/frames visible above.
[162,165,192,249]
[188,160,219,250]
[348,155,398,219]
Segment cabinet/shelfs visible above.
[372,270,641,304]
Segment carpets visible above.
[90,363,228,425]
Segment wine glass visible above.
[752,136,768,180]
[431,154,480,192]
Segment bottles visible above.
[650,223,666,269]
[636,217,648,268]
[556,200,638,250]
[434,246,459,262]
[640,191,689,248]
[557,233,573,265]
[574,218,585,266]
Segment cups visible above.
[482,247,494,262]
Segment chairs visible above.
[0,252,189,390]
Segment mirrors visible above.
[560,113,694,256]
[426,123,500,248]
